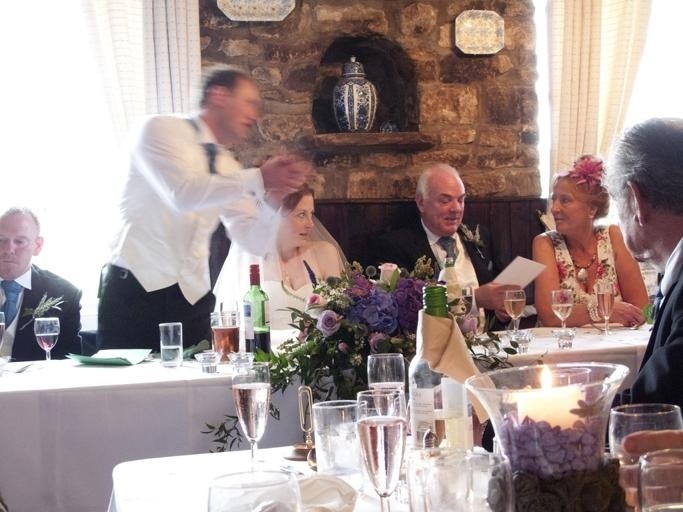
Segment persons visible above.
[532,154,650,327]
[0,207,83,361]
[212,182,350,353]
[432,118,683,454]
[366,162,526,331]
[95,69,313,354]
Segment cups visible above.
[514,342,527,356]
[155,310,254,372]
[556,335,573,352]
[421,361,683,512]
[207,471,301,512]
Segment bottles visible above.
[409,285,475,512]
[243,264,271,365]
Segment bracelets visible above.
[589,304,602,322]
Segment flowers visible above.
[200,255,545,453]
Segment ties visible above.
[1,281,21,328]
[438,236,458,267]
[204,143,217,174]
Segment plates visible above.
[216,0,296,22]
[453,10,506,57]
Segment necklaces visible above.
[570,250,596,281]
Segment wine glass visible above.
[551,289,575,338]
[34,318,61,370]
[596,282,615,340]
[232,352,407,512]
[502,289,526,340]
[0,312,6,376]
